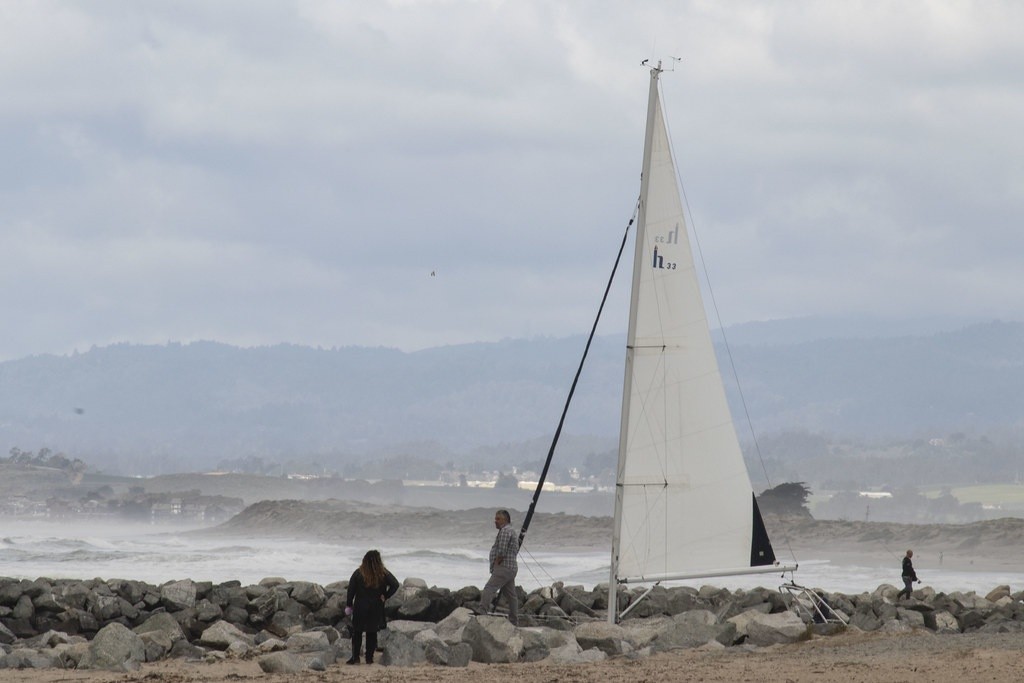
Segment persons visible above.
[897,550,917,600]
[811,592,828,623]
[345,550,399,665]
[468,510,518,626]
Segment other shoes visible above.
[366,656,374,664]
[346,657,360,664]
[469,611,480,617]
[897,594,900,601]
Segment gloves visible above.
[345,607,352,616]
